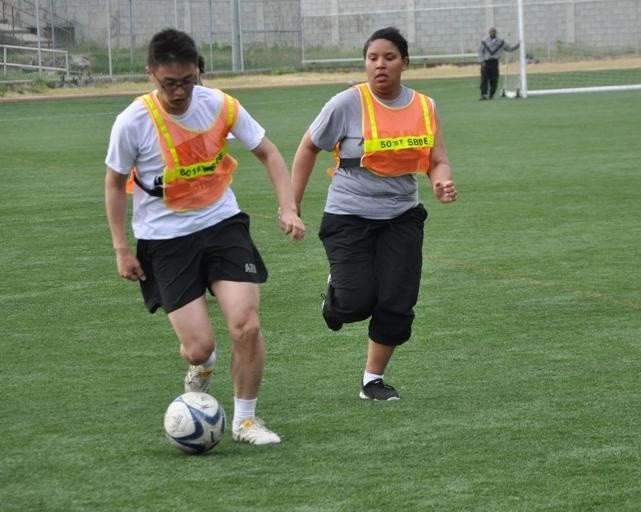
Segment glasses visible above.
[150,69,198,94]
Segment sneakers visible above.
[232,418,281,446]
[321,274,343,332]
[359,378,399,401]
[184,364,213,393]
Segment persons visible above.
[479,26,521,100]
[102,26,306,446]
[277,27,459,401]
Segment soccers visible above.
[164,390,228,453]
